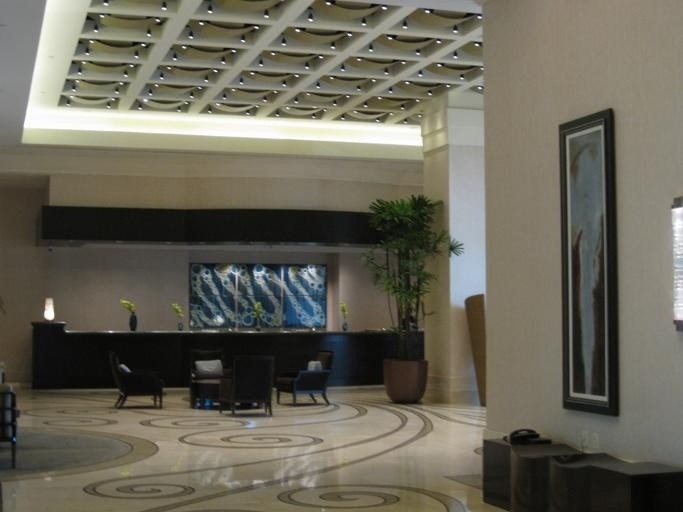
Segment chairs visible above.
[189,349,224,375]
[111,363,164,408]
[0,380,16,469]
[220,356,272,416]
[273,343,333,406]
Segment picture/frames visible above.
[557,107,619,416]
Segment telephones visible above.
[509,429,540,444]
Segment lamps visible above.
[43,297,56,322]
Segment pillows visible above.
[119,365,130,373]
[308,360,321,370]
[195,359,224,376]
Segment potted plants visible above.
[360,195,465,403]
[172,303,184,330]
[254,302,263,332]
[338,300,348,332]
[120,297,137,332]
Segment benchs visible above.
[482,437,682,512]
